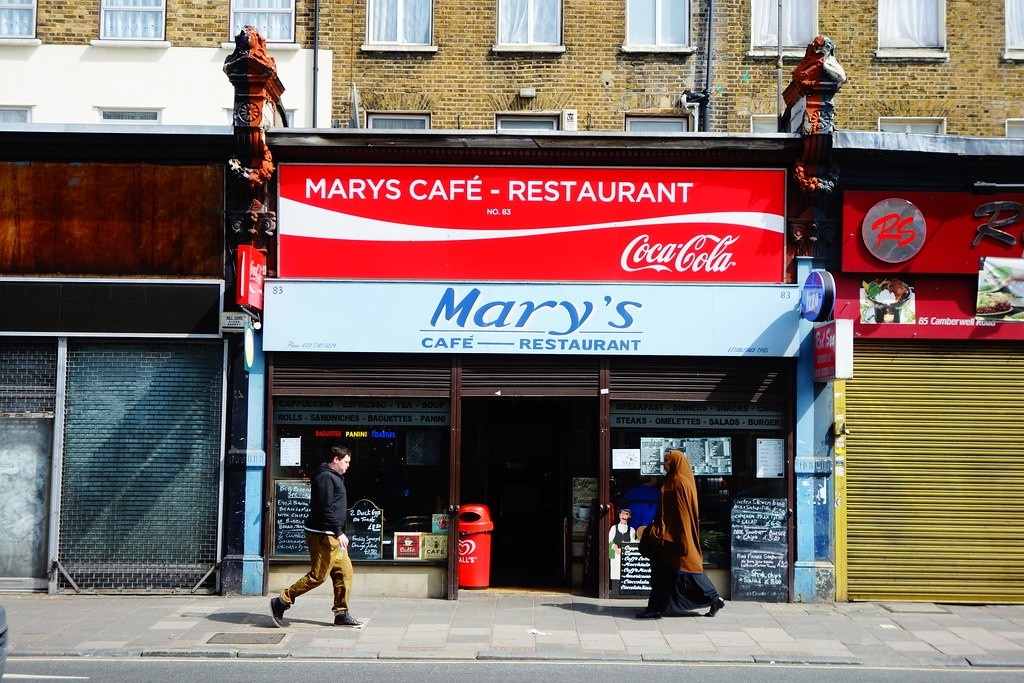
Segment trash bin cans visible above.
[458,503,494,590]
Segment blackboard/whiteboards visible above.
[731,497,790,604]
[271,477,311,559]
[619,541,653,597]
[345,500,383,560]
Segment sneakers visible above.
[334,610,364,628]
[269,597,291,627]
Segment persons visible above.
[635,450,726,619]
[268,445,363,627]
[608,509,635,555]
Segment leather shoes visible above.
[634,609,662,620]
[705,597,725,617]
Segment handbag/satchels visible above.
[638,519,682,564]
